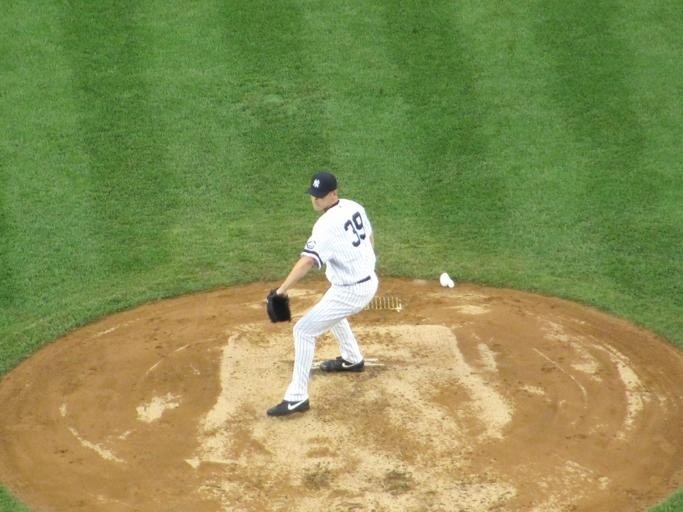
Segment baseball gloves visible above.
[269,290,289,322]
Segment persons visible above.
[266,172,380,414]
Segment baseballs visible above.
[440,272,454,288]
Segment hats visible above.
[303,171,337,198]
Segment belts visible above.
[335,276,370,287]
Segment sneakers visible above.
[319,356,364,372]
[267,399,310,416]
